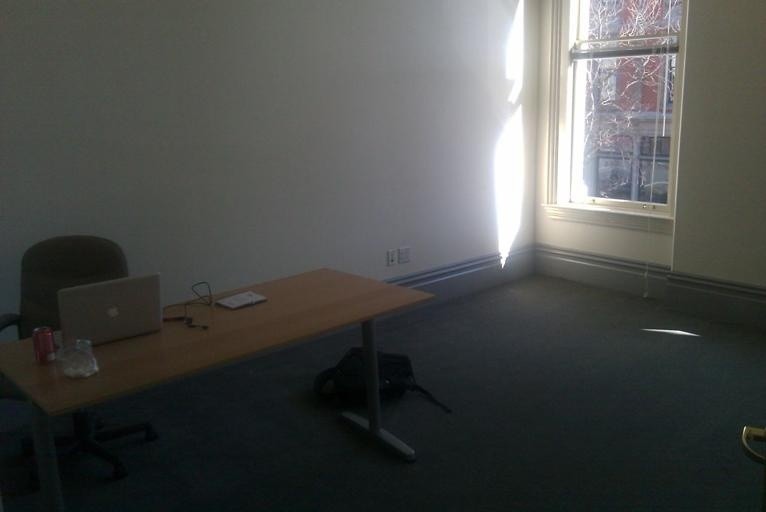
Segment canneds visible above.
[32,326,56,364]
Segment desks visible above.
[0,266,436,512]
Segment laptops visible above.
[53,272,161,348]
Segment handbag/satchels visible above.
[313,347,412,404]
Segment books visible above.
[215,289,267,312]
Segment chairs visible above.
[0,236,159,493]
